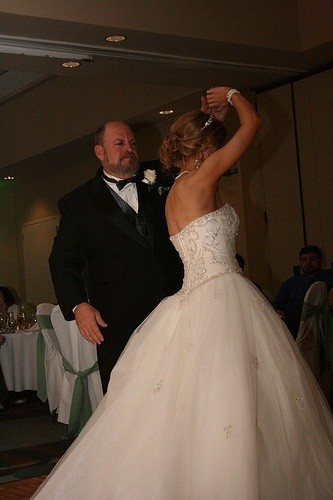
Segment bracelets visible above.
[227,89,240,106]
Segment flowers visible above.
[141,168,157,192]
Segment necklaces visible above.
[175,171,190,180]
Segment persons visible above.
[272,245,333,341]
[235,253,271,304]
[24,86,333,500]
[47,96,229,395]
[0,335,18,416]
[0,287,17,318]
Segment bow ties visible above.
[101,169,140,191]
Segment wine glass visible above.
[18,314,24,333]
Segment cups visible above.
[30,313,37,324]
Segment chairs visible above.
[36,303,102,429]
[296,281,327,372]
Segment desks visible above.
[0,330,42,404]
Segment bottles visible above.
[20,305,27,322]
[8,311,17,333]
[0,312,8,333]
